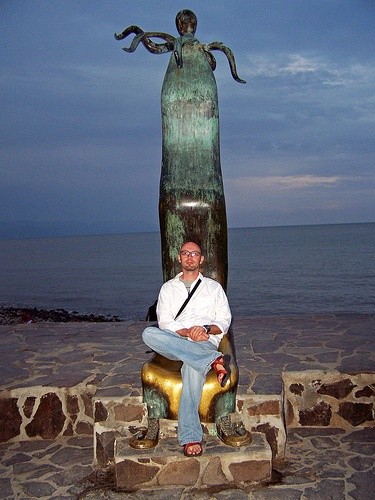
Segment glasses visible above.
[180,249,202,257]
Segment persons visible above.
[142,242,232,457]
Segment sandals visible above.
[184,441,203,457]
[211,354,232,387]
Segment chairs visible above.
[129,331,253,449]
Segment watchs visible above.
[203,325,211,334]
